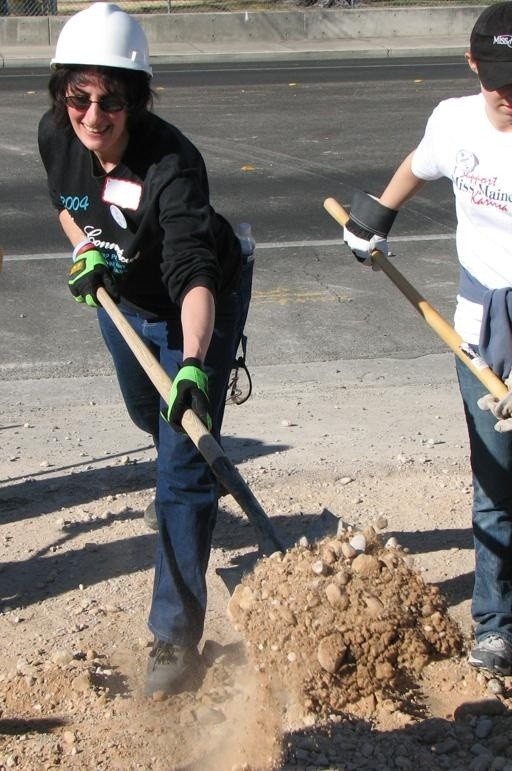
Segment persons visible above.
[38,2,252,698]
[341,3,512,677]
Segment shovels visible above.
[95,286,462,717]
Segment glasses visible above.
[64,95,128,112]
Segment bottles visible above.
[234,223,255,265]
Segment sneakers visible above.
[469,635,511,675]
[144,500,160,530]
[144,638,198,695]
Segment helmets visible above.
[50,2,153,77]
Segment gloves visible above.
[477,372,512,433]
[68,239,121,307]
[343,190,398,271]
[159,357,212,432]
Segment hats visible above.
[470,1,512,91]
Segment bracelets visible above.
[72,240,90,261]
